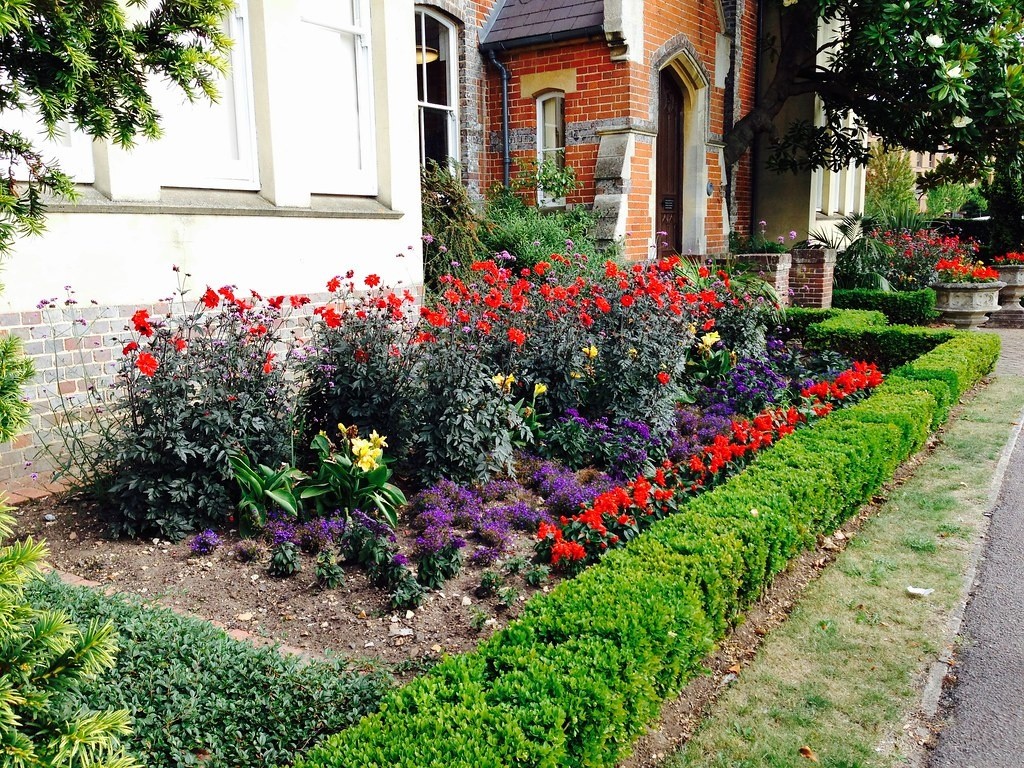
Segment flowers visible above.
[937,257,1001,284]
[989,250,1024,265]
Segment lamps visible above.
[416,45,439,64]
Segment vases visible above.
[986,265,1024,321]
[930,282,1008,330]
